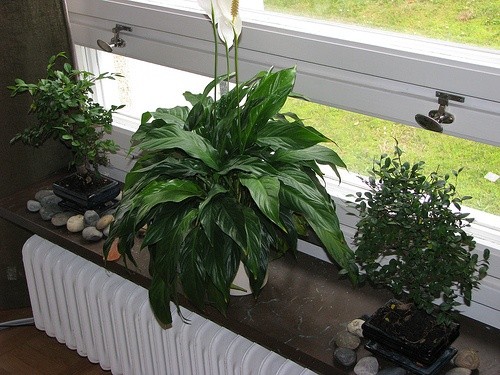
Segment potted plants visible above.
[6,48,120,214]
[340,136,490,375]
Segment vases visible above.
[228,258,269,299]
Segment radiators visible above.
[21,232,320,375]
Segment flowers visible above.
[100,0,360,333]
